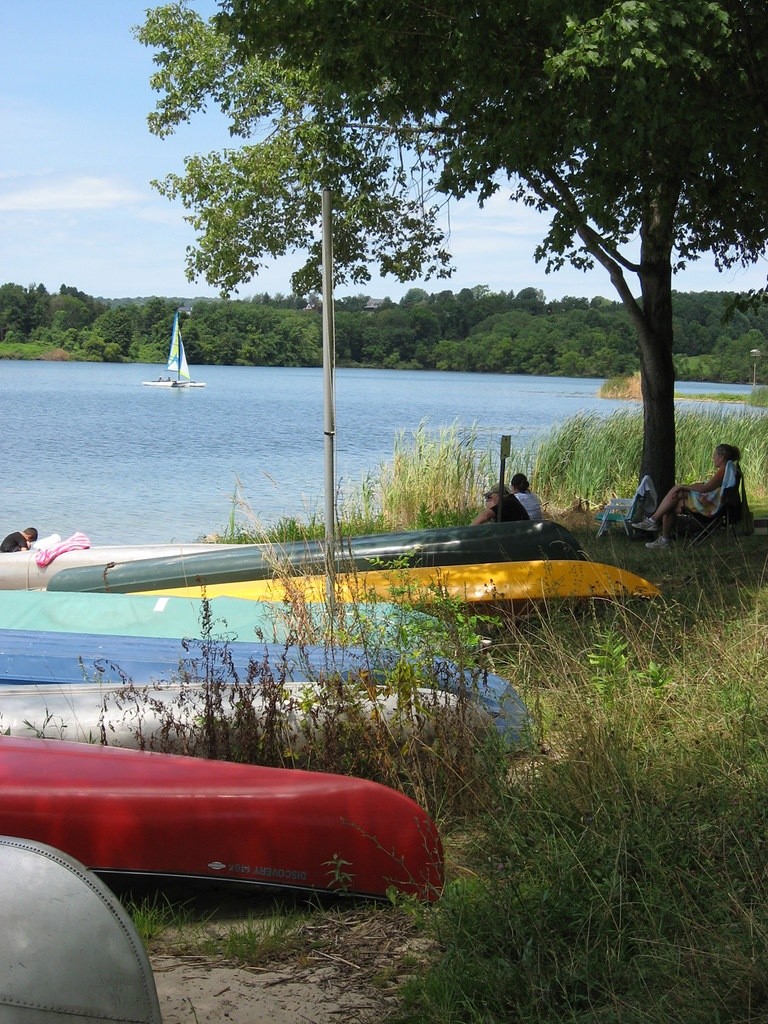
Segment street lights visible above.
[749,348,761,387]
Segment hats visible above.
[485,483,508,496]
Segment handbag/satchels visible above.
[729,506,755,538]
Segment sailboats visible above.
[142,311,206,388]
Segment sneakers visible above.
[631,518,657,531]
[646,536,669,549]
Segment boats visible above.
[0,520,665,1024]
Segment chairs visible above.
[595,475,649,538]
[677,459,739,549]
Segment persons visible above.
[158,376,171,382]
[632,444,740,549]
[0,527,38,553]
[469,483,531,526]
[510,473,543,520]
